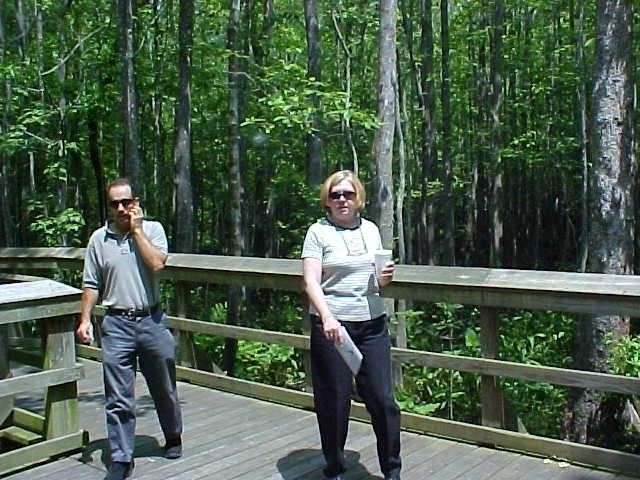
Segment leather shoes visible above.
[104,460,134,480]
[165,437,182,458]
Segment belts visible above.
[111,306,156,317]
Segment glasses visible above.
[330,191,356,200]
[110,199,132,209]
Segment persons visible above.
[76,178,183,480]
[300,169,402,479]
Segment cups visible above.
[375,250,392,277]
